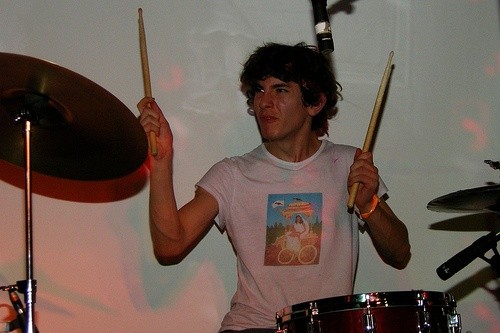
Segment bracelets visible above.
[359,193,381,219]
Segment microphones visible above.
[312,0,335,54]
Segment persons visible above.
[136,41,412,333]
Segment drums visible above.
[275,290,461,333]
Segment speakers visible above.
[9,291,40,333]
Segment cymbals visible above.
[426,185,500,212]
[0,52,148,180]
[428,211,500,231]
